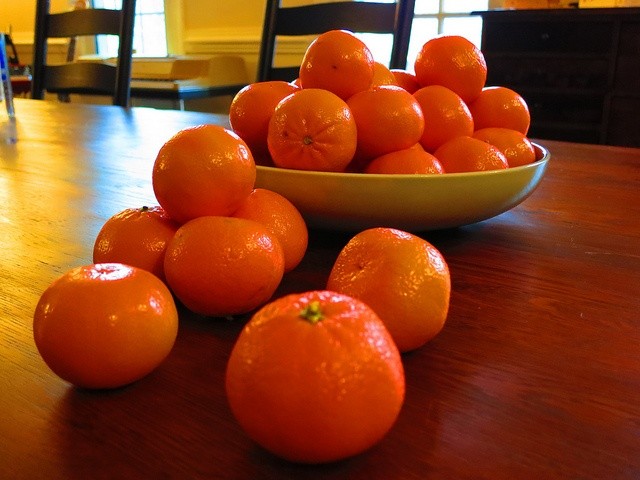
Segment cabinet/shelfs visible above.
[471,5,639,148]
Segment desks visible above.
[0,97,640,480]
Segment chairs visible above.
[256,0,416,83]
[32,0,136,108]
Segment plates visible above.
[255,141,551,233]
